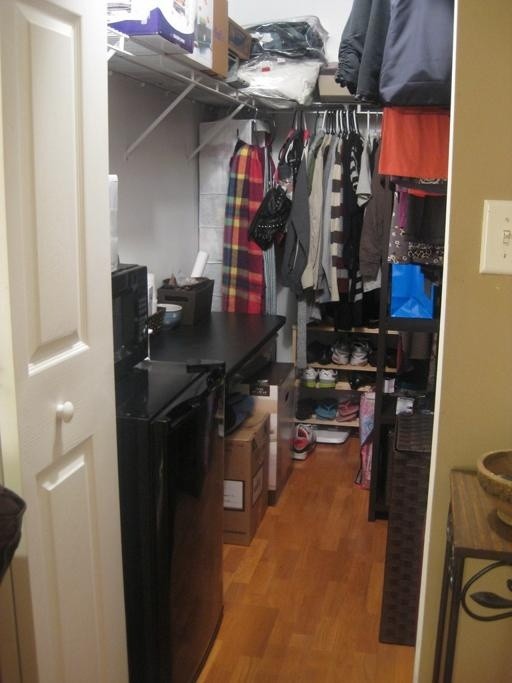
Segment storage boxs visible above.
[390,262,436,319]
[318,69,362,103]
[378,414,432,647]
[224,410,270,546]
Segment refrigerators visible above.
[115,360,224,683]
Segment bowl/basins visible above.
[476,448,512,527]
[157,303,183,331]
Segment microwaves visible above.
[111,263,149,375]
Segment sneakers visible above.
[292,424,317,460]
[298,336,396,393]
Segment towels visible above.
[376,105,451,179]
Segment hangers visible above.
[277,106,383,168]
[107,0,252,77]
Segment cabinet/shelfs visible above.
[367,178,447,522]
[227,361,295,508]
[290,325,414,428]
[196,118,271,311]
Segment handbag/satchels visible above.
[246,185,292,252]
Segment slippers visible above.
[296,396,358,423]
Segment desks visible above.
[113,311,288,683]
[433,470,512,683]
